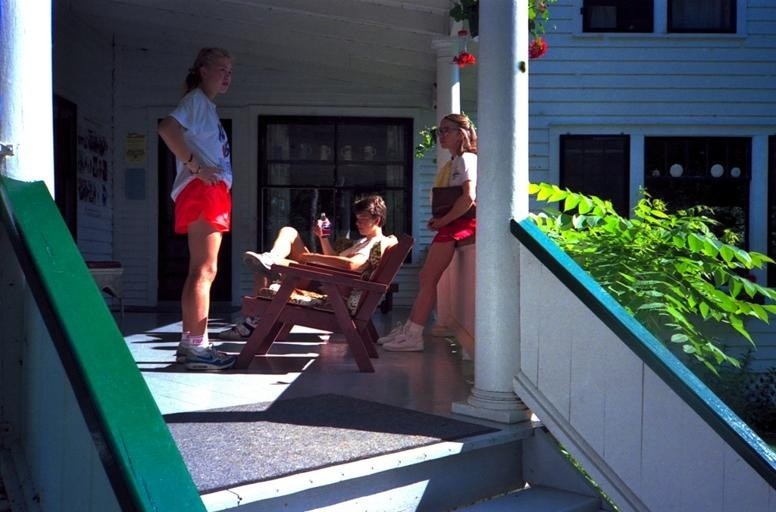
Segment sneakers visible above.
[218,321,256,341]
[242,251,279,281]
[175,343,238,370]
[376,325,424,352]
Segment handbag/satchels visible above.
[432,186,476,219]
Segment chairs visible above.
[231,234,415,372]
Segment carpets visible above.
[159,391,501,496]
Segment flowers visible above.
[451,0,548,70]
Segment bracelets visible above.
[182,151,195,167]
[190,165,202,175]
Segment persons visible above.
[155,45,238,371]
[377,111,478,352]
[219,194,389,339]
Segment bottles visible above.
[320,213,332,238]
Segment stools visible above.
[85,260,125,319]
